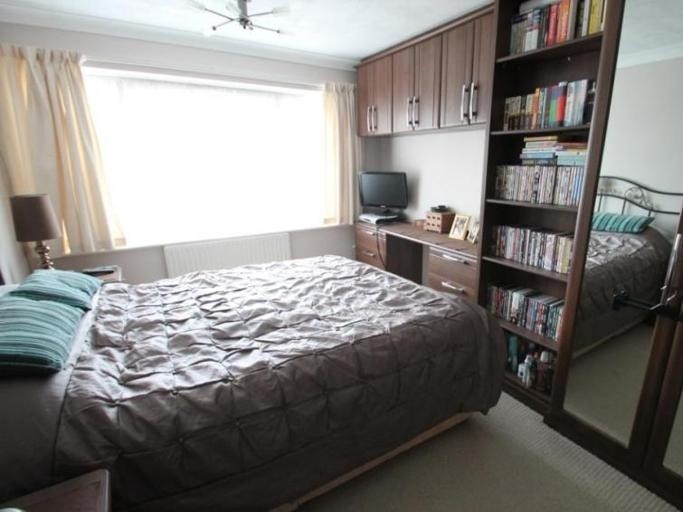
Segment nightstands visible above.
[88,263,121,284]
[0,468,112,511]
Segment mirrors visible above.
[560,2,683,447]
[663,391,682,479]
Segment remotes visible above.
[82,268,114,274]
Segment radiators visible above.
[160,232,294,279]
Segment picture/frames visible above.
[465,217,480,244]
[447,212,472,241]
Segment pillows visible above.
[0,295,87,373]
[8,268,104,310]
[590,212,656,234]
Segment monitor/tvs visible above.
[358,171,408,208]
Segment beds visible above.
[0,252,507,511]
[569,175,683,369]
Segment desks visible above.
[352,220,481,305]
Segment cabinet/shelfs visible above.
[439,3,494,135]
[480,1,625,416]
[542,1,683,511]
[392,29,441,139]
[355,51,391,138]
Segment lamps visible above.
[189,1,289,37]
[9,192,60,269]
